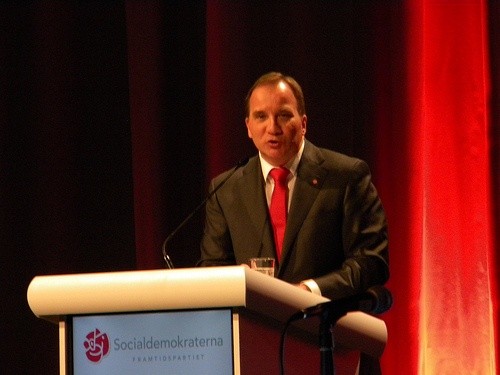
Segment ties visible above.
[269,167,290,264]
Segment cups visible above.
[250,256,275,279]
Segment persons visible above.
[199,71,390,311]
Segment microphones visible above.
[288,286,393,322]
[162,155,248,269]
